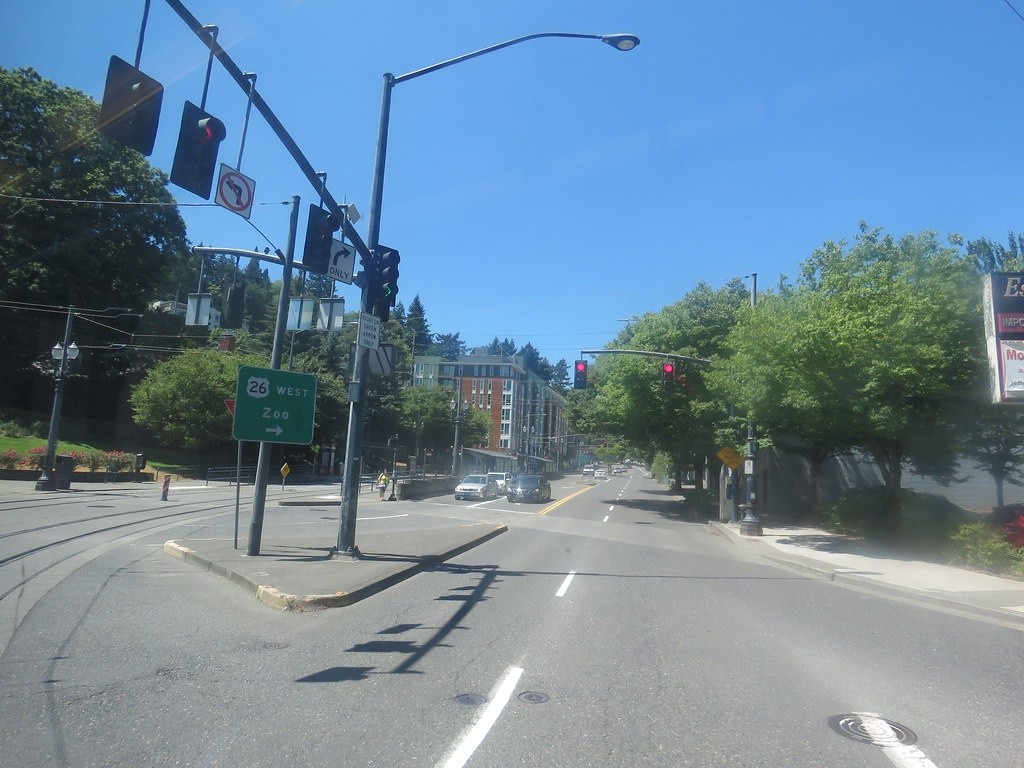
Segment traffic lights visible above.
[662,363,675,391]
[365,245,401,323]
[573,360,588,390]
[169,100,226,200]
[96,55,164,155]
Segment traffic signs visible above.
[232,366,317,444]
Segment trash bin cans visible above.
[55,454,73,490]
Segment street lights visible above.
[35,305,81,490]
[326,29,643,553]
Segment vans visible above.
[583,465,594,475]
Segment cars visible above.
[621,468,627,472]
[454,473,499,501]
[614,468,622,474]
[486,472,513,496]
[507,476,551,503]
[595,470,606,479]
